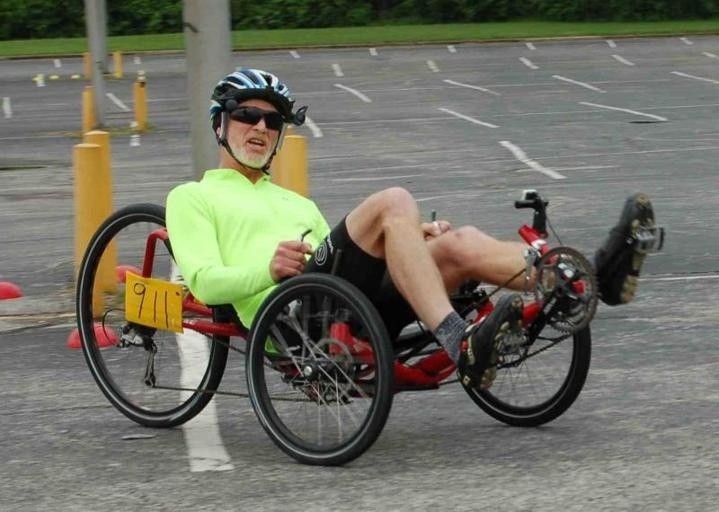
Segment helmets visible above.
[207,67,297,128]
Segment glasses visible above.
[224,104,285,131]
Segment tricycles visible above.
[75,190,666,466]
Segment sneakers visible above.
[456,290,525,393]
[593,190,655,306]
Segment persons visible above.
[162,64,667,394]
